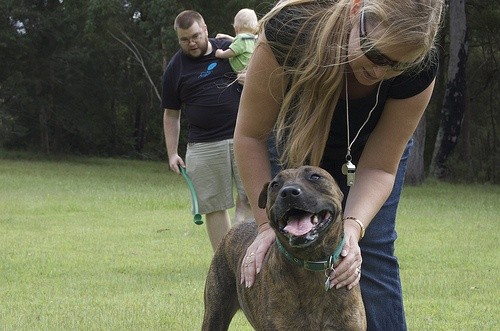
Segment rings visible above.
[357,269,361,273]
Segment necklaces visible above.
[342,67,384,187]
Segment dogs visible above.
[200,163,368,331]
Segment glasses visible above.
[360,7,423,71]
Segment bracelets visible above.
[341,216,365,240]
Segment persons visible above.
[232,0,444,331]
[215,7,258,95]
[162,9,252,253]
